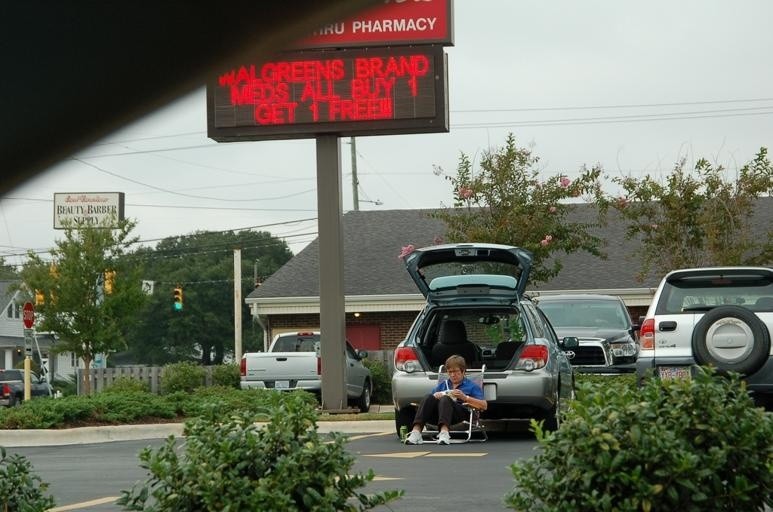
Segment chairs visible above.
[422,364,488,444]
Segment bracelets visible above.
[465,394,470,403]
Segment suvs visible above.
[532,293,639,383]
[633,264,772,409]
[385,242,581,436]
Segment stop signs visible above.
[21,299,35,329]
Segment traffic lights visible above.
[173,288,184,311]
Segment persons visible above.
[400,354,489,447]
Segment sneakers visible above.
[404,429,425,446]
[435,430,451,445]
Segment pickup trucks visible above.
[0,368,54,409]
[237,331,374,417]
